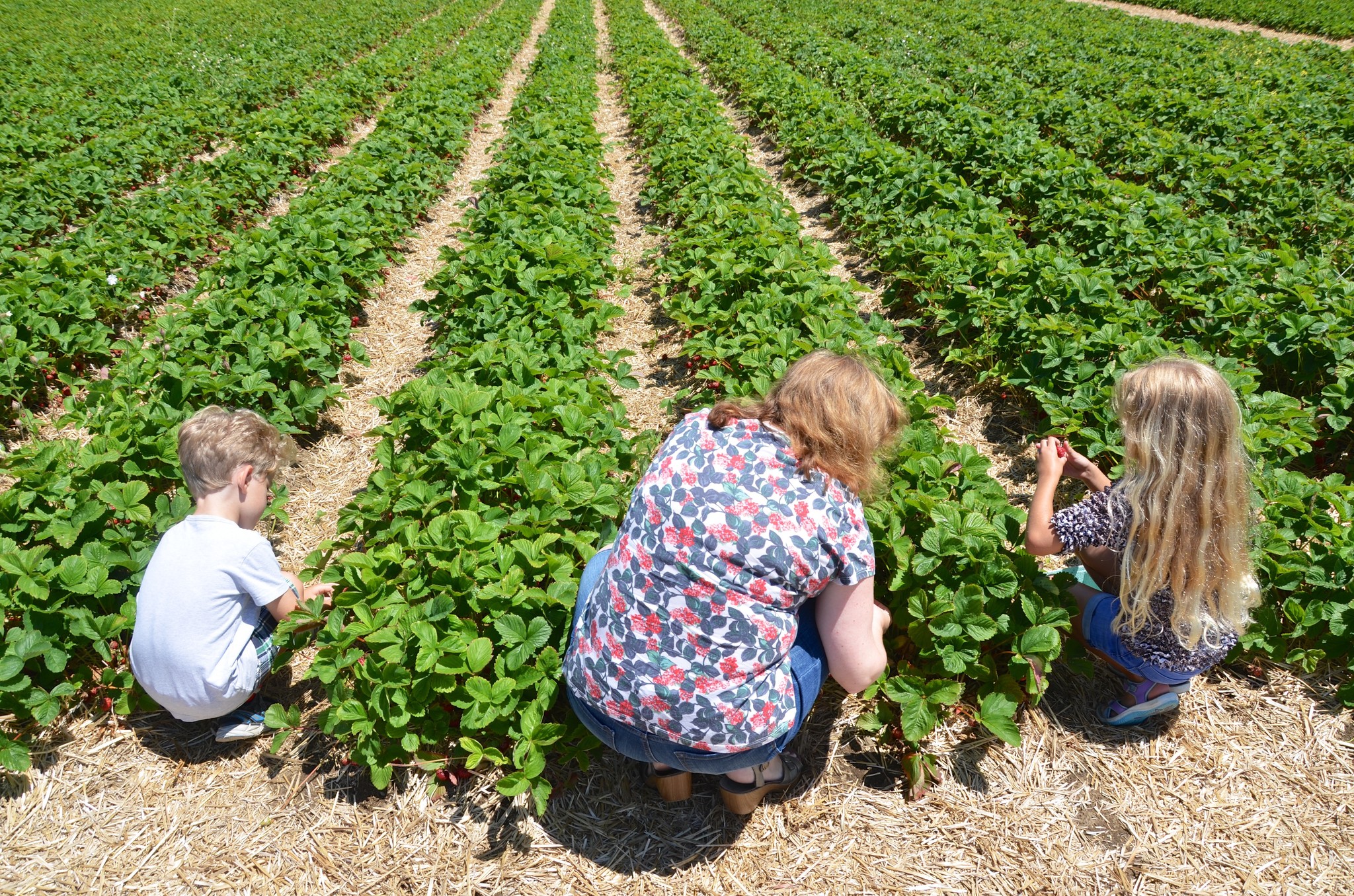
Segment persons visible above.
[560,347,912,817]
[1024,353,1269,726]
[128,403,340,743]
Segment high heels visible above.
[720,752,804,815]
[643,761,692,802]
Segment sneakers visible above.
[214,693,282,742]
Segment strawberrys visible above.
[0,30,1332,781]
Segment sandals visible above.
[1096,680,1179,726]
[1168,681,1190,694]
